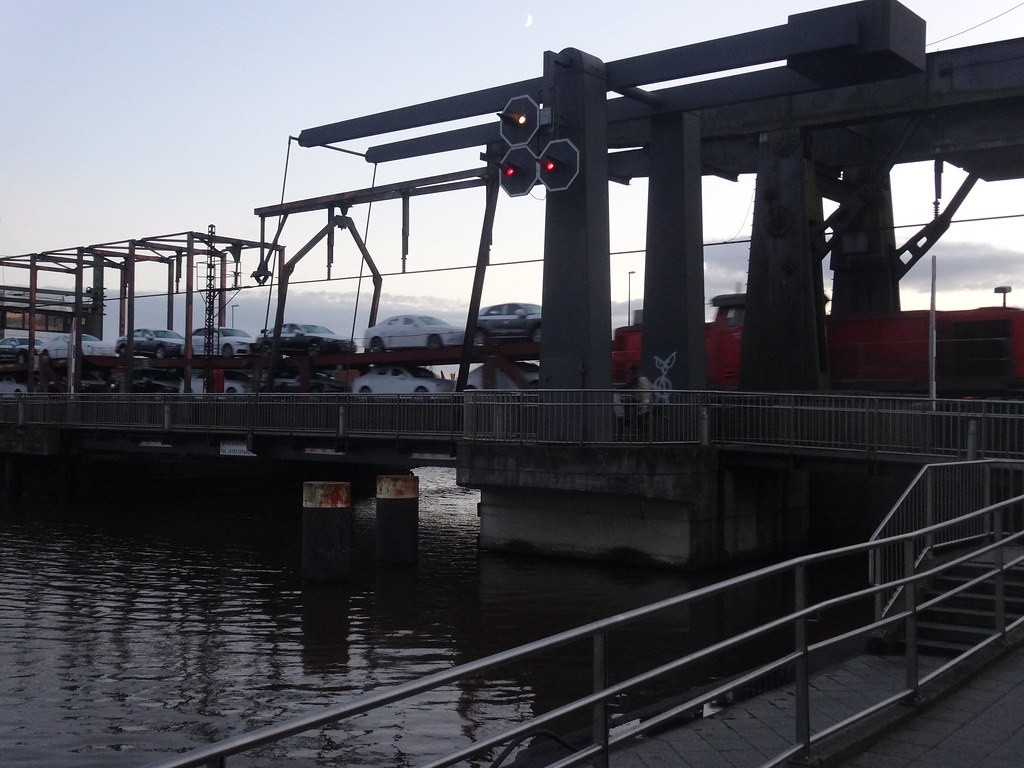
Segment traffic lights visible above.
[494,145,537,198]
[533,139,580,193]
[496,95,538,147]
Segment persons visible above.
[626,364,651,439]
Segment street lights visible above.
[995,287,1011,306]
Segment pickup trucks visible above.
[608,290,1024,396]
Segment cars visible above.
[474,303,544,342]
[179,370,258,399]
[114,328,186,358]
[257,323,357,355]
[181,326,255,357]
[36,333,114,358]
[351,366,456,399]
[32,372,107,400]
[0,337,43,363]
[253,369,351,396]
[453,362,540,391]
[0,375,28,400]
[112,368,181,400]
[364,313,466,354]
